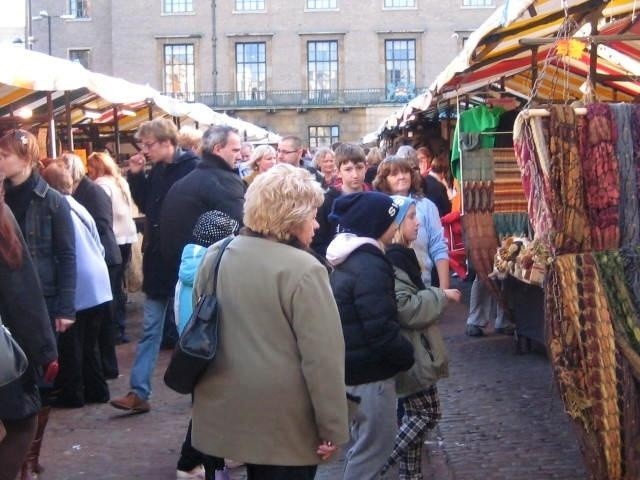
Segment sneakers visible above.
[496,325,513,334]
[465,324,483,336]
[176,465,205,480]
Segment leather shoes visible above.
[111,392,150,411]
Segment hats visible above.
[397,144,419,168]
[328,191,416,239]
[192,211,239,247]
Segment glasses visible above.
[140,141,157,148]
[277,149,296,154]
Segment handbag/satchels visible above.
[0,317,28,387]
[125,233,144,293]
[164,294,218,396]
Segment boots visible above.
[19,406,50,480]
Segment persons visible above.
[464,270,543,338]
[0,114,463,480]
